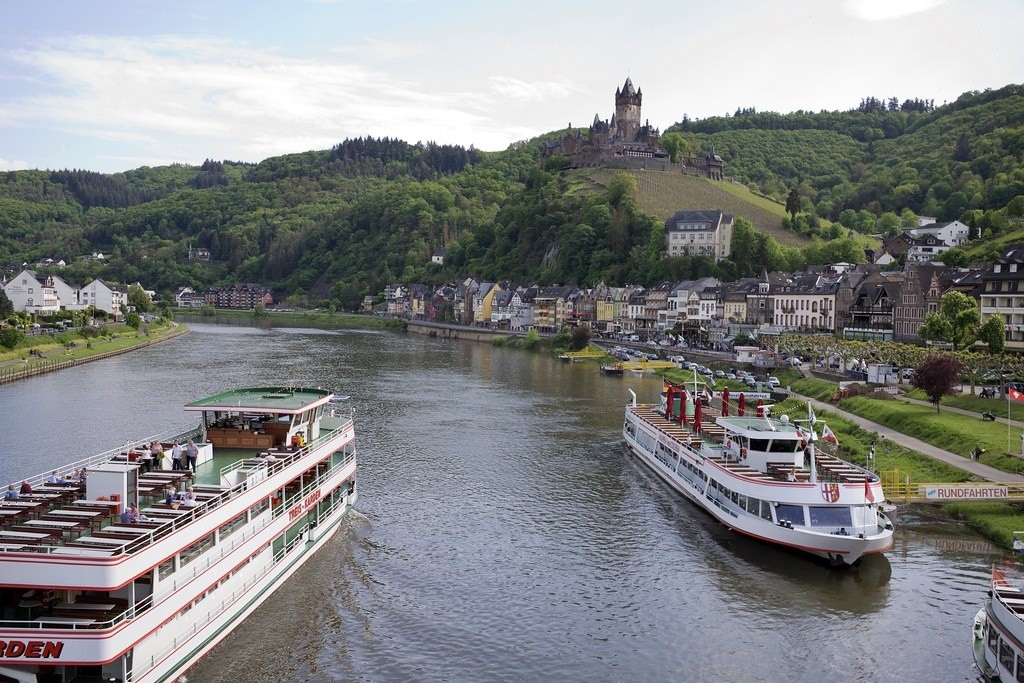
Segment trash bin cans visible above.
[296,431,304,443]
[291,436,300,445]
[110,493,120,514]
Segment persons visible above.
[4,480,34,499]
[29,349,41,357]
[71,340,76,348]
[646,357,648,362]
[278,441,299,451]
[885,444,889,453]
[129,440,164,472]
[608,349,611,355]
[121,503,141,525]
[979,386,995,398]
[685,433,691,451]
[987,410,995,421]
[970,446,981,461]
[254,451,277,463]
[787,468,799,482]
[166,486,197,505]
[49,467,88,487]
[931,396,937,406]
[172,440,199,475]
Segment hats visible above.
[186,487,193,491]
[125,507,132,512]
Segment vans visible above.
[631,335,639,342]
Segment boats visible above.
[0,384,360,683]
[559,355,573,361]
[623,367,895,570]
[970,531,1024,683]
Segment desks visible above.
[0,447,233,557]
[635,408,736,447]
[252,448,297,466]
[711,453,869,484]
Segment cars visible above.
[40,326,68,335]
[677,342,688,349]
[696,345,708,350]
[264,308,296,312]
[681,362,781,388]
[785,358,802,366]
[666,356,685,364]
[607,346,659,362]
[646,341,657,347]
[621,336,630,341]
[658,340,671,347]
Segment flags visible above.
[1009,388,1024,401]
[822,425,839,446]
[865,478,874,502]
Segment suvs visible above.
[897,367,914,379]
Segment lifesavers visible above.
[95,496,110,516]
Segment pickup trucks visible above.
[997,381,1024,394]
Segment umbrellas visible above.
[722,386,764,417]
[665,385,702,437]
